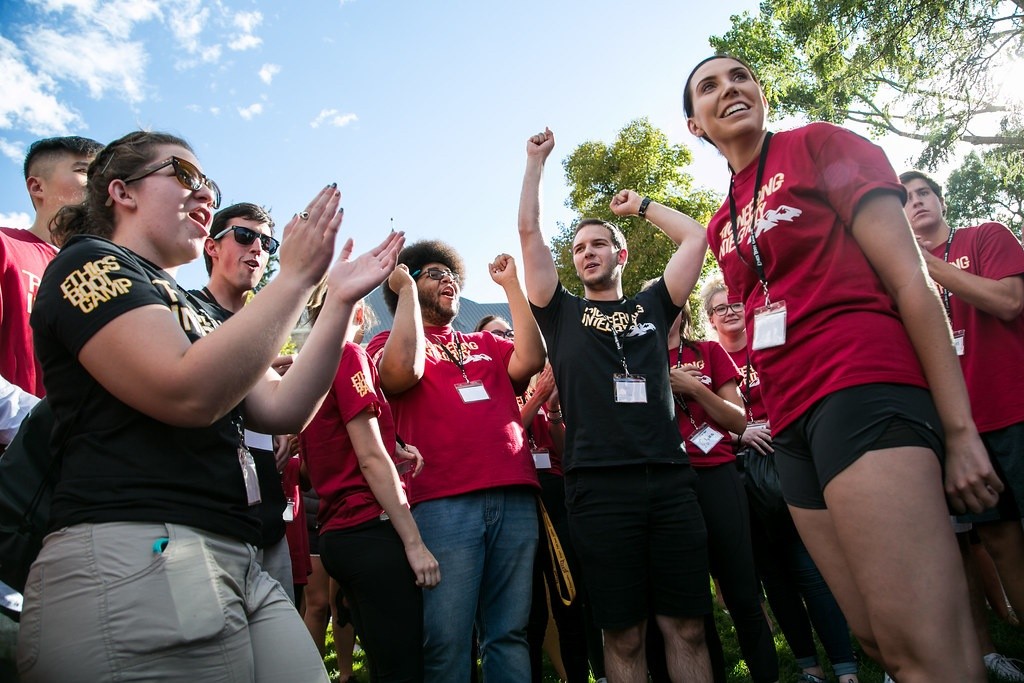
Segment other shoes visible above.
[1007,612,1020,625]
[983,653,1024,683]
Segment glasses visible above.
[213,225,280,255]
[491,330,515,342]
[710,302,744,317]
[416,270,459,284]
[105,156,222,210]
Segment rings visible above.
[300,212,310,219]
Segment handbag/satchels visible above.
[744,445,788,514]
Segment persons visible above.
[684,57,1024,683]
[367,125,730,683]
[0,130,442,683]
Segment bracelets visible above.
[638,197,651,218]
[546,409,563,423]
[738,435,743,444]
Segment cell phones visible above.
[151,537,168,556]
